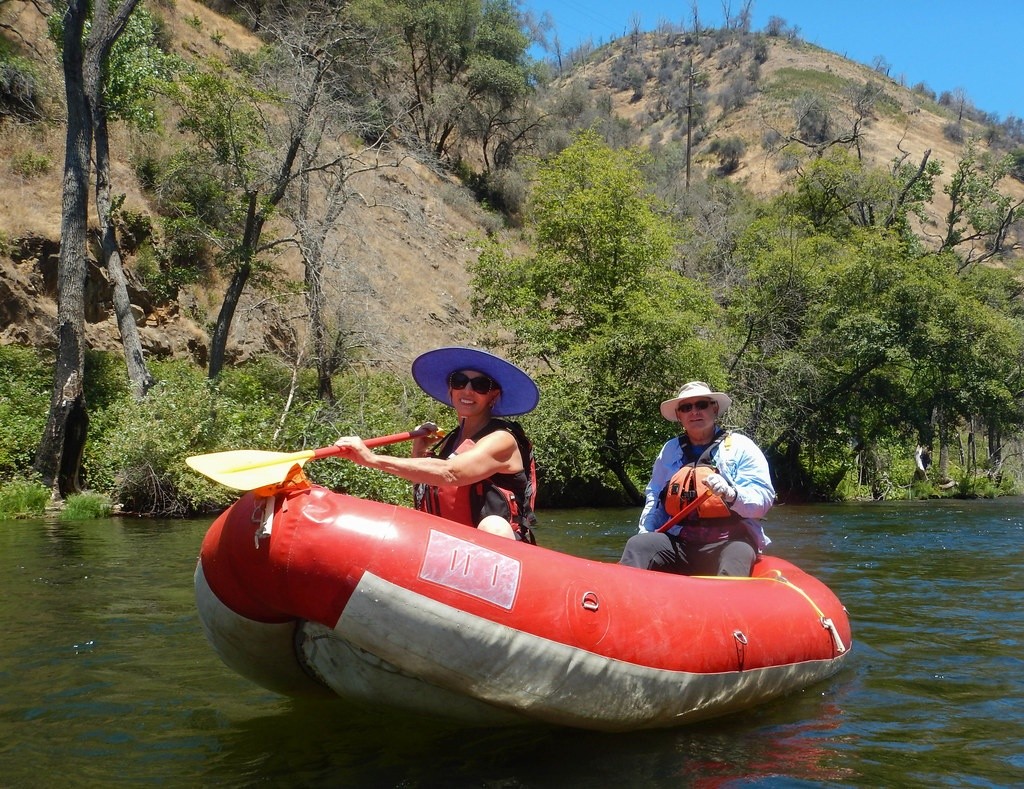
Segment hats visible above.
[660,381,732,422]
[411,347,540,417]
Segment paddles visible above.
[655,490,715,531]
[183,426,447,492]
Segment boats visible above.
[195,464,851,733]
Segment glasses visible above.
[449,372,498,395]
[677,400,715,413]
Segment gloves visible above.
[701,474,736,502]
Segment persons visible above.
[619,380,776,577]
[334,346,540,544]
